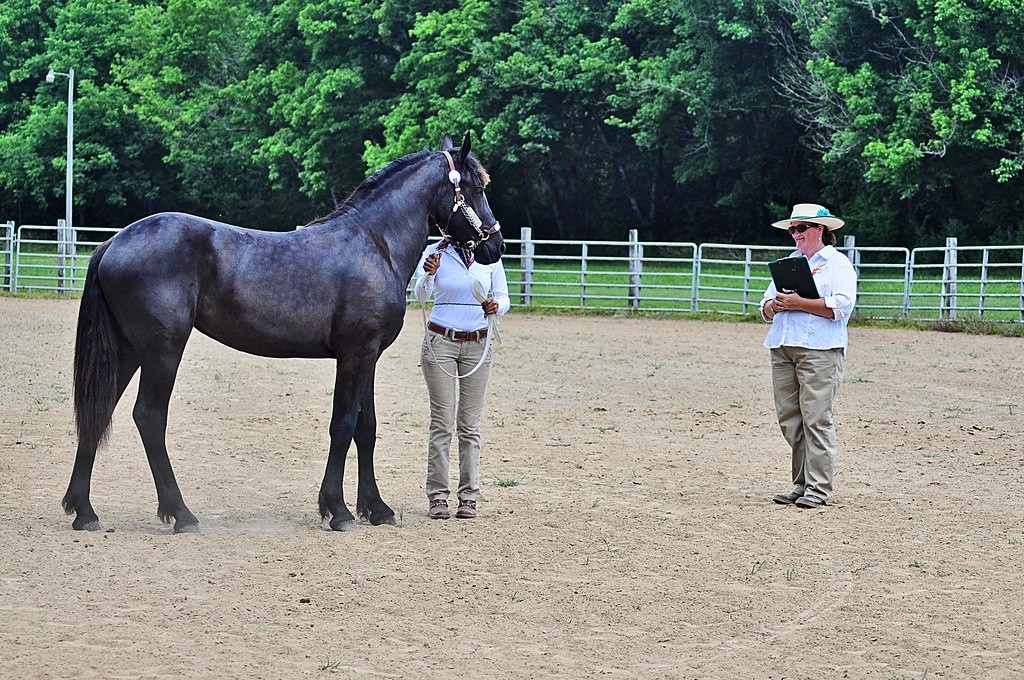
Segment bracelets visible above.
[769,302,777,315]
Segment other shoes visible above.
[773,492,803,504]
[429,499,450,518]
[457,499,477,517]
[795,495,825,508]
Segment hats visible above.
[772,204,845,232]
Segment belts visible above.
[427,321,488,341]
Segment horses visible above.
[61,130,509,532]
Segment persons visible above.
[759,203,858,508]
[414,231,511,519]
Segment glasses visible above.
[788,224,813,234]
